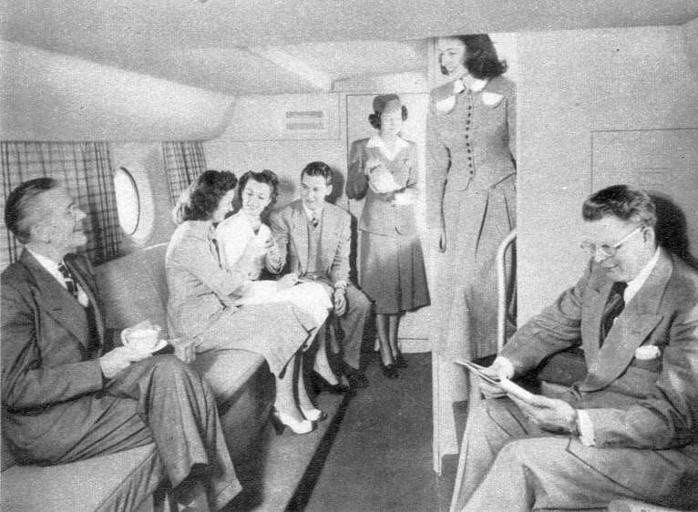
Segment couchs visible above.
[0,242,275,512]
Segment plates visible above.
[126,339,168,355]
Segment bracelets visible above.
[567,411,579,439]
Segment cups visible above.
[125,328,159,352]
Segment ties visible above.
[599,281,631,357]
[57,262,78,302]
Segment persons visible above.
[346,93,430,383]
[1,176,244,512]
[445,182,698,510]
[424,35,519,372]
[214,168,346,395]
[162,168,327,437]
[266,158,373,392]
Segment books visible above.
[454,359,548,406]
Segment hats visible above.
[373,94,402,115]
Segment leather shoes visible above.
[379,348,408,380]
[341,360,369,390]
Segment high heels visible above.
[268,405,314,437]
[296,404,325,424]
[311,369,348,395]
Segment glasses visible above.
[580,226,643,258]
[33,201,81,228]
